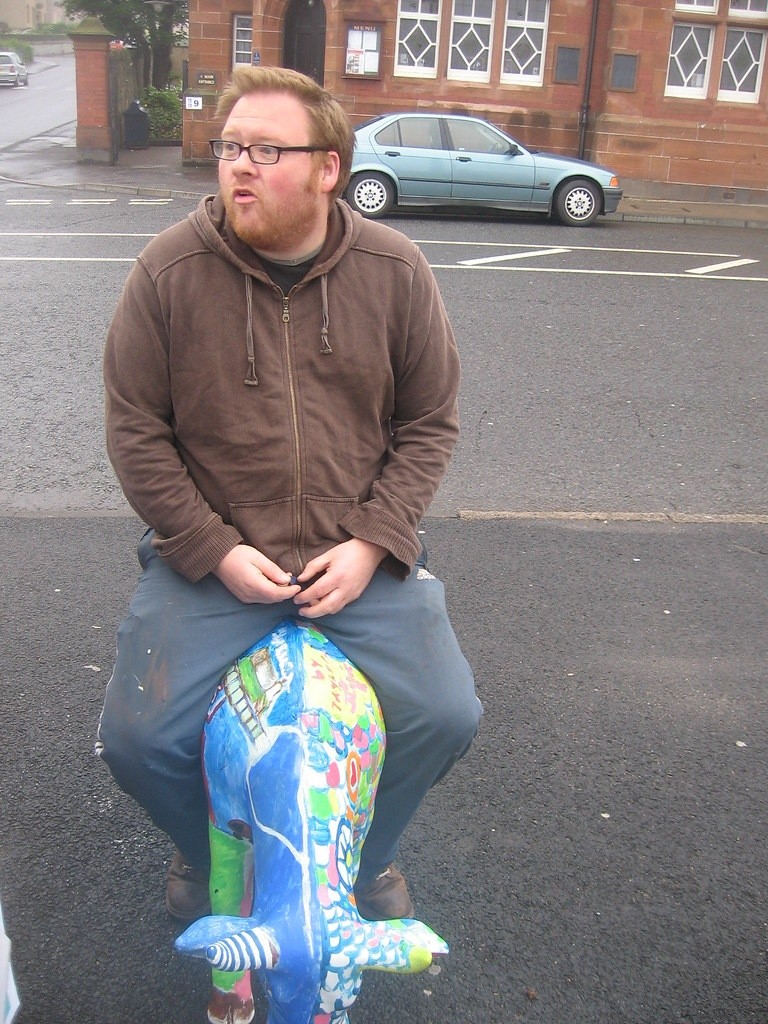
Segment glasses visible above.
[208,138,331,164]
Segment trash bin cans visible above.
[124,99,149,151]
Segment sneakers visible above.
[354,863,416,920]
[165,849,211,919]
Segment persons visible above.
[95,67,486,924]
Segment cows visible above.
[172,614,452,1024]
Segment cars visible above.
[342,114,622,228]
[0,52,29,88]
